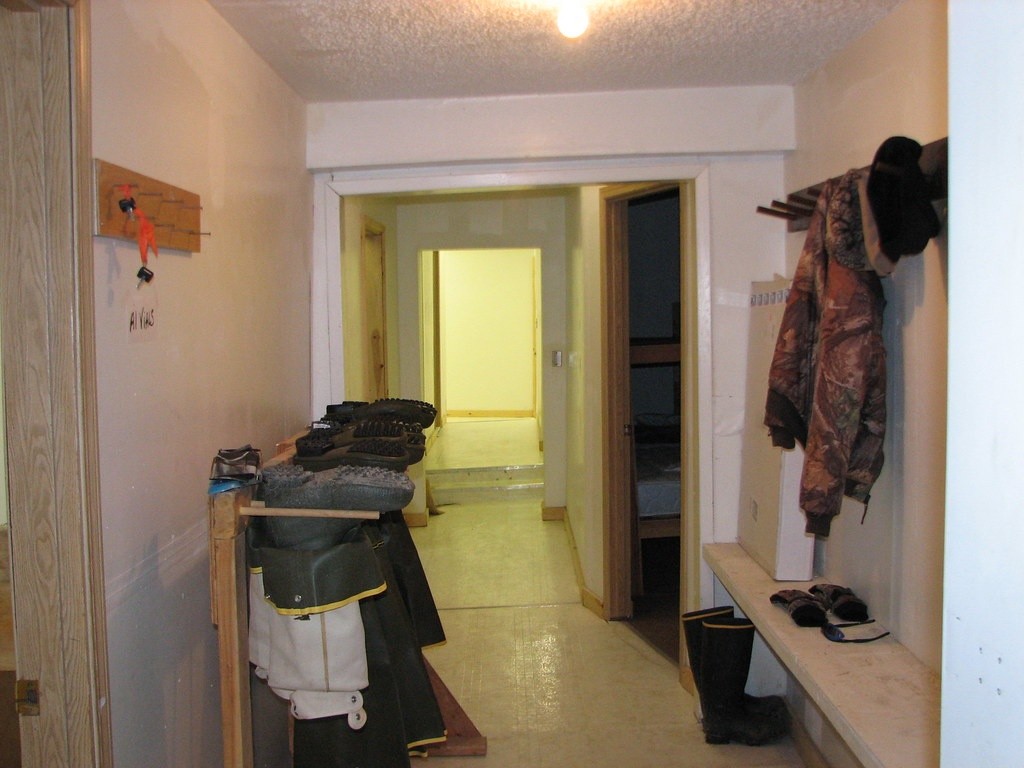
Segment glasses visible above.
[820,620,888,643]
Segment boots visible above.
[683,605,782,717]
[254,398,448,768]
[698,618,787,747]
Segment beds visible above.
[637,439,681,584]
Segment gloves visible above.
[809,583,869,624]
[772,588,831,627]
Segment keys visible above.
[135,267,154,289]
[119,197,136,221]
